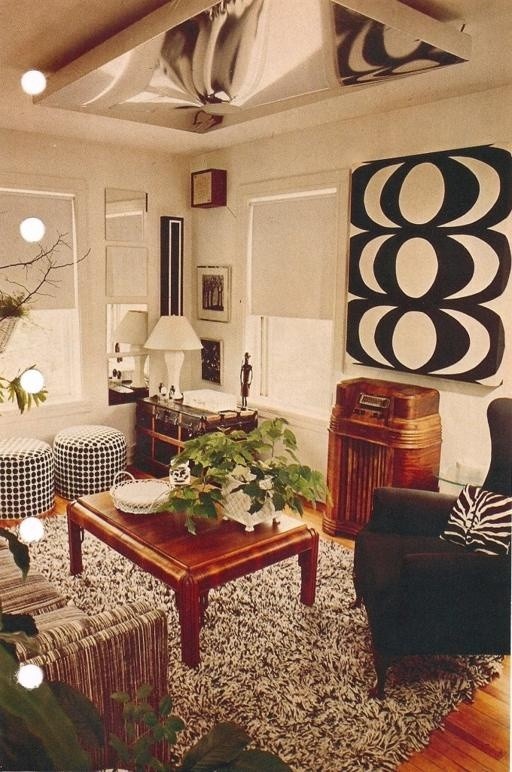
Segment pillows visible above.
[439,484,511,557]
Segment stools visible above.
[1,436,54,521]
[52,424,127,500]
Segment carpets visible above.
[0,513,503,772]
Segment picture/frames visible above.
[105,247,148,296]
[106,189,146,243]
[197,266,231,322]
[201,336,224,386]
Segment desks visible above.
[135,391,257,477]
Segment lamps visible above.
[113,311,145,389]
[146,315,205,401]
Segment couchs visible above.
[1,538,169,771]
[351,398,512,698]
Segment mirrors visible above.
[106,305,146,406]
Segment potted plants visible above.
[157,418,333,535]
